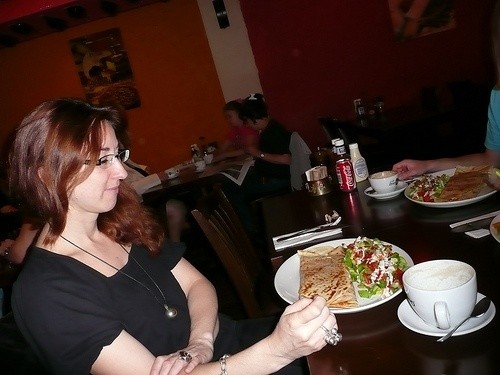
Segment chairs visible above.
[191,183,284,319]
[292,133,307,190]
[132,172,160,196]
[318,118,354,148]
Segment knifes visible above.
[277,224,347,241]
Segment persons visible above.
[0,97,339,375]
[239,92,293,196]
[392,0,500,191]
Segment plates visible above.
[273,238,415,314]
[397,292,495,337]
[364,181,408,200]
[489,214,500,243]
[403,168,498,208]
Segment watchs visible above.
[260,153,264,157]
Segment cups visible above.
[402,260,477,332]
[164,168,176,179]
[368,171,398,193]
[194,160,206,172]
[305,175,332,196]
[203,154,213,164]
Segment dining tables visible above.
[142,156,243,204]
[255,179,500,375]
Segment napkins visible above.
[464,227,490,237]
[271,224,341,251]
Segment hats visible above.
[239,93,266,119]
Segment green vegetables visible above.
[344,239,405,298]
[410,173,449,200]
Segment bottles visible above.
[190,144,202,160]
[330,138,370,192]
[354,99,367,118]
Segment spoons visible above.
[436,296,491,341]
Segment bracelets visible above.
[219,354,226,375]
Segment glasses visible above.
[83,149,130,169]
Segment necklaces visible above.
[47,221,178,318]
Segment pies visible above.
[297,245,358,308]
[440,165,491,201]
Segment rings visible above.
[179,351,192,362]
[321,326,343,346]
